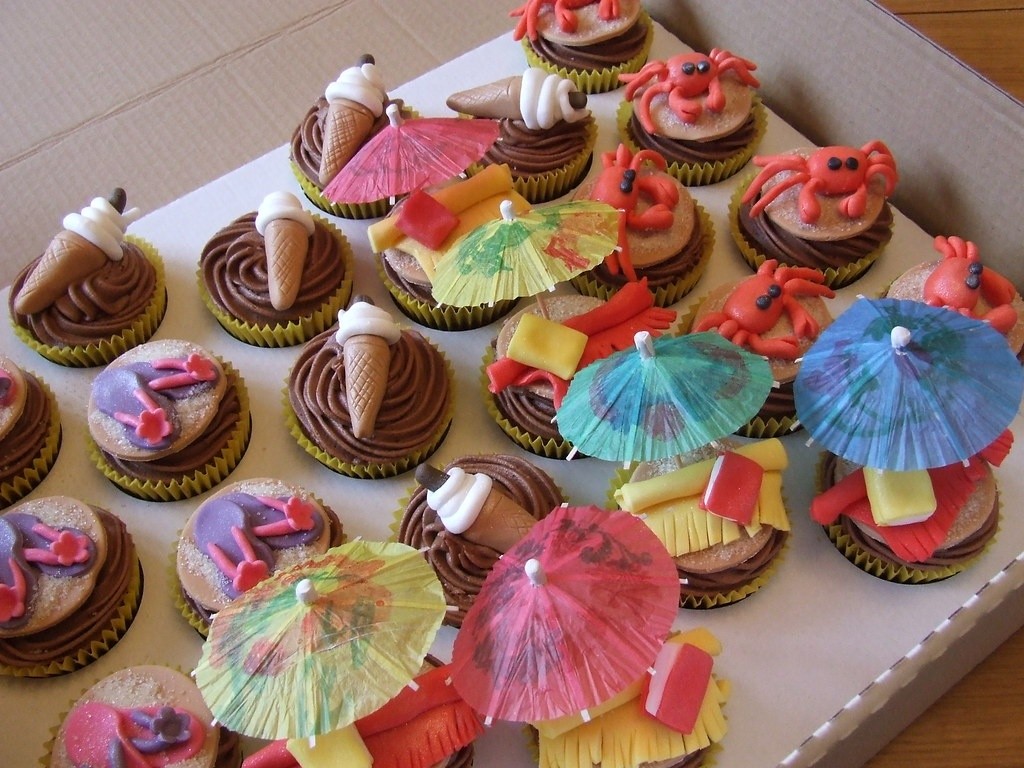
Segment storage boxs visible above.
[0,0,1024,768]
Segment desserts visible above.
[0,0,1024,768]
